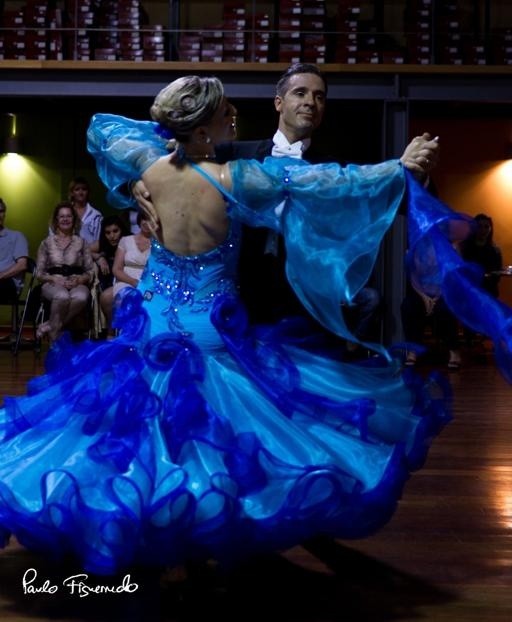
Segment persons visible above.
[0,62,512,607]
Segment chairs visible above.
[0,264,119,356]
